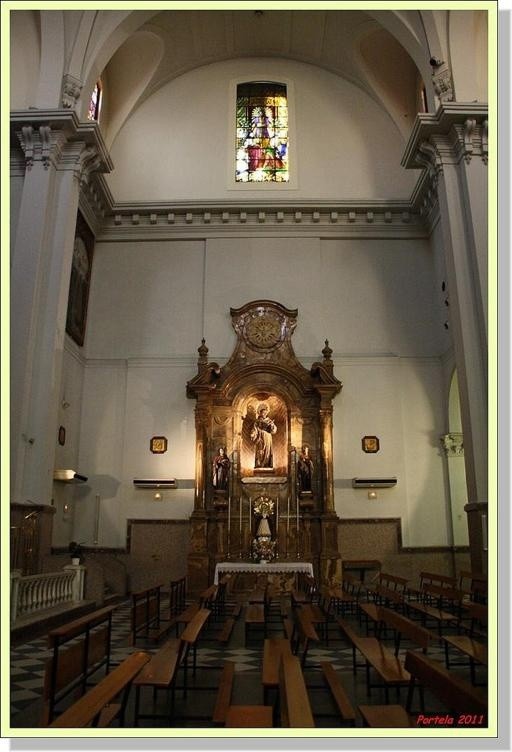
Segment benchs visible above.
[49,571,487,728]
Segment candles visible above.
[228,496,299,532]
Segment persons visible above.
[297,445,315,494]
[252,109,286,169]
[211,447,230,489]
[250,409,277,469]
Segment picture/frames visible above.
[362,436,379,453]
[150,437,167,454]
[59,426,65,446]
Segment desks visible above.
[342,560,382,590]
[212,562,315,601]
